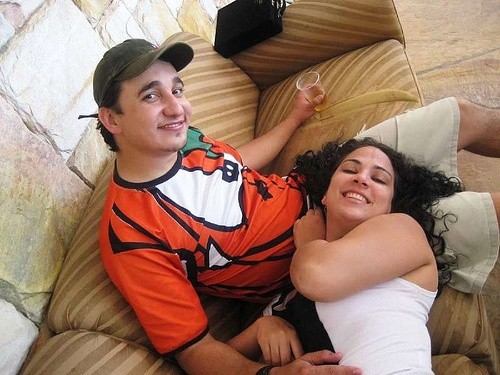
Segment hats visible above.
[78,38,194,120]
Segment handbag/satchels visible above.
[214,0,286,59]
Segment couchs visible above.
[19,0,498,375]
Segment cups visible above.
[296,71,328,112]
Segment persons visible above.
[77,38,500,375]
[223,138,466,375]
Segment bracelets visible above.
[256,365,275,374]
